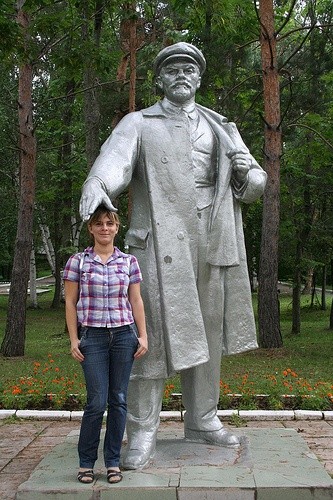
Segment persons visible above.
[77,43,269,472]
[62,204,150,484]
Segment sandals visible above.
[107,470,124,484]
[77,470,94,483]
